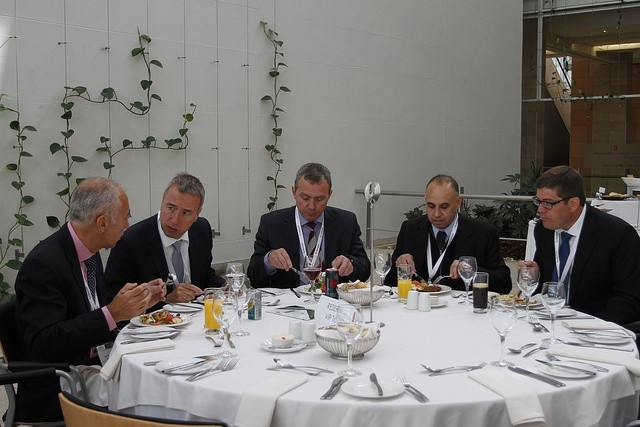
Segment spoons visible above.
[528,321,542,332]
[273,358,334,376]
[421,362,486,374]
[261,300,281,306]
[506,343,537,353]
[156,273,178,290]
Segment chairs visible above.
[0,370,76,427]
[0,301,91,427]
[59,393,220,427]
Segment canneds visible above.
[248,290,262,320]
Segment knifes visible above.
[535,359,596,376]
[326,378,348,399]
[370,373,382,396]
[539,316,596,320]
[161,358,215,373]
[413,271,428,285]
[121,330,182,334]
[564,341,634,354]
[290,286,301,299]
[523,347,545,357]
[165,301,202,309]
[508,365,566,388]
[570,331,633,339]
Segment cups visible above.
[288,320,302,342]
[472,272,488,314]
[301,323,317,348]
[205,288,222,334]
[398,263,412,304]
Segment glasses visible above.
[532,197,573,210]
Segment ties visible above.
[172,241,185,285]
[84,255,96,301]
[307,222,326,272]
[437,232,447,250]
[558,233,573,305]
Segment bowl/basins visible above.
[336,284,382,306]
[315,326,381,359]
[272,333,295,347]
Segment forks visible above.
[320,376,344,400]
[433,275,459,285]
[265,368,328,377]
[222,325,236,349]
[394,371,428,403]
[545,352,609,373]
[185,357,229,381]
[190,358,238,382]
[205,336,223,347]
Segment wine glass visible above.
[229,278,251,336]
[541,282,566,349]
[458,256,478,307]
[336,304,364,377]
[212,291,238,350]
[491,297,515,366]
[518,268,539,320]
[303,255,322,303]
[374,253,394,288]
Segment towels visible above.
[562,319,636,341]
[550,344,640,377]
[234,373,308,427]
[467,367,545,426]
[100,337,174,381]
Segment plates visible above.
[198,297,207,304]
[260,336,306,353]
[601,194,628,200]
[534,306,577,317]
[128,327,179,340]
[576,330,631,344]
[340,375,404,399]
[530,356,597,379]
[257,288,286,296]
[163,303,203,312]
[129,312,193,326]
[157,358,214,375]
[410,283,452,294]
[298,284,329,296]
[428,297,447,308]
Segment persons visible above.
[383,174,513,295]
[104,170,228,329]
[515,164,640,357]
[246,162,372,289]
[3,176,168,422]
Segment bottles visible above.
[407,290,418,309]
[419,292,431,312]
